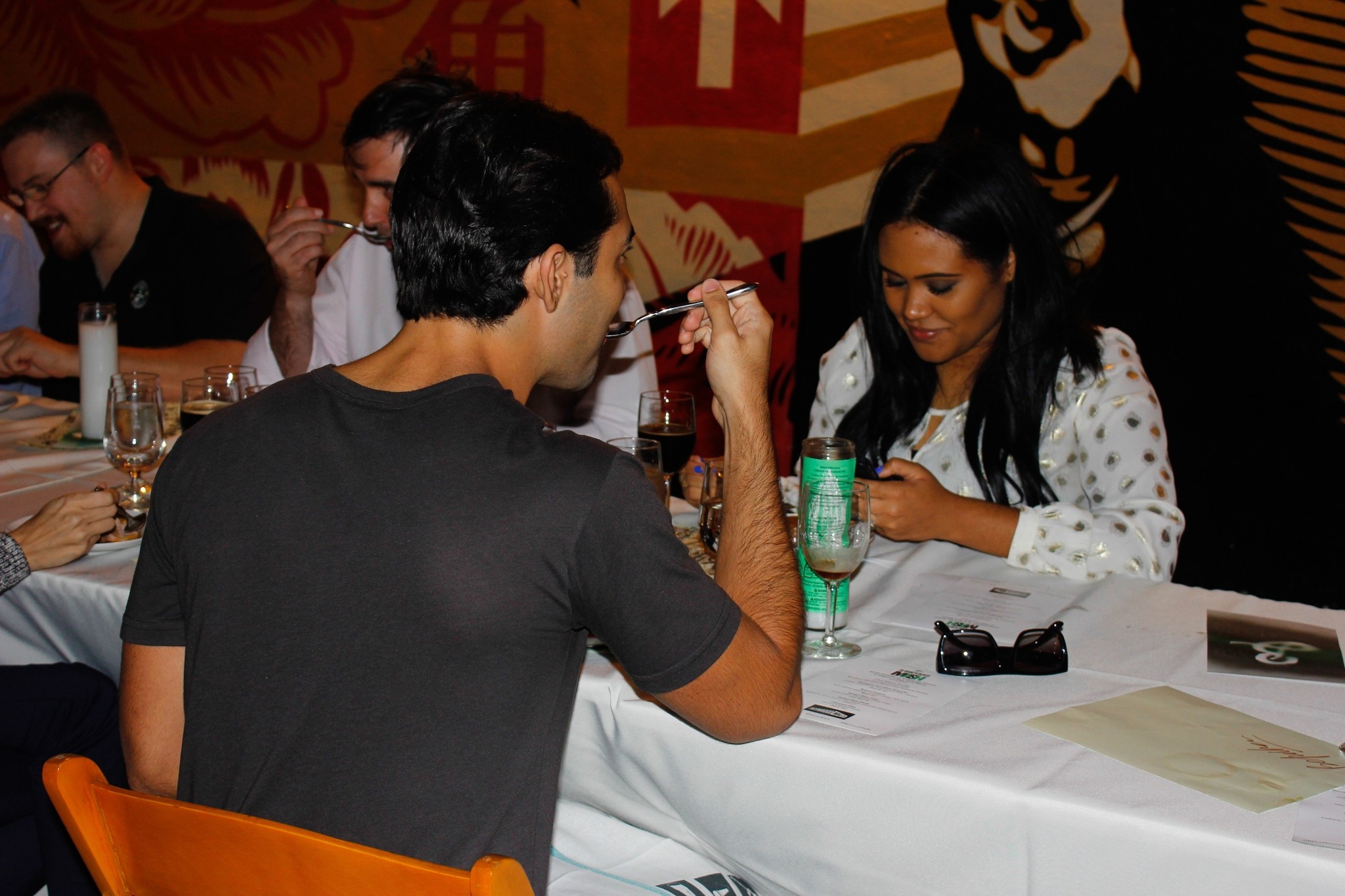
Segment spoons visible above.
[315,217,391,244]
[605,280,759,338]
[94,486,147,533]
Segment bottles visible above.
[796,437,856,631]
[78,301,119,441]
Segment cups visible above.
[179,377,240,433]
[204,365,258,404]
[698,461,726,560]
[607,437,665,507]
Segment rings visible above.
[679,471,690,488]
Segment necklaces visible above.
[935,377,971,409]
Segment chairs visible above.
[43,751,527,896]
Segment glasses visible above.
[935,620,1068,676]
[5,145,91,208]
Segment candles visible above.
[78,317,121,443]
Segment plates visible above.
[780,476,800,517]
[7,509,147,553]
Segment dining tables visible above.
[0,386,1345,896]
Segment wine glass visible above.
[797,478,872,659]
[637,389,697,511]
[102,372,166,510]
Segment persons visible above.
[232,46,666,460]
[1,479,134,896]
[111,86,810,894]
[678,134,1186,587]
[0,196,49,399]
[0,87,280,407]
